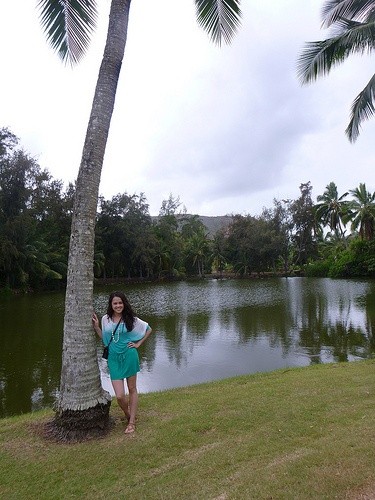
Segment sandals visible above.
[124,423,136,434]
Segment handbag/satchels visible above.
[102,347,109,359]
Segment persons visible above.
[92,291,153,433]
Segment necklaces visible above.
[111,316,120,343]
